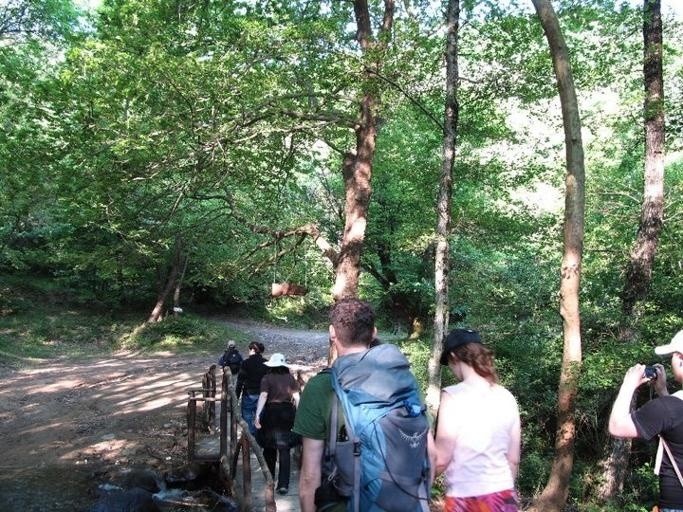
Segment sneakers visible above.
[275,483,289,494]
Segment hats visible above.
[439,327,483,366]
[654,328,683,356]
[262,352,292,369]
[226,339,236,349]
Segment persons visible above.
[424,328,522,512]
[608,329,683,511]
[234,341,267,436]
[218,339,243,389]
[290,296,436,512]
[254,353,300,493]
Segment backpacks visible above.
[329,343,434,512]
[222,350,241,375]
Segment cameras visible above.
[642,367,658,380]
